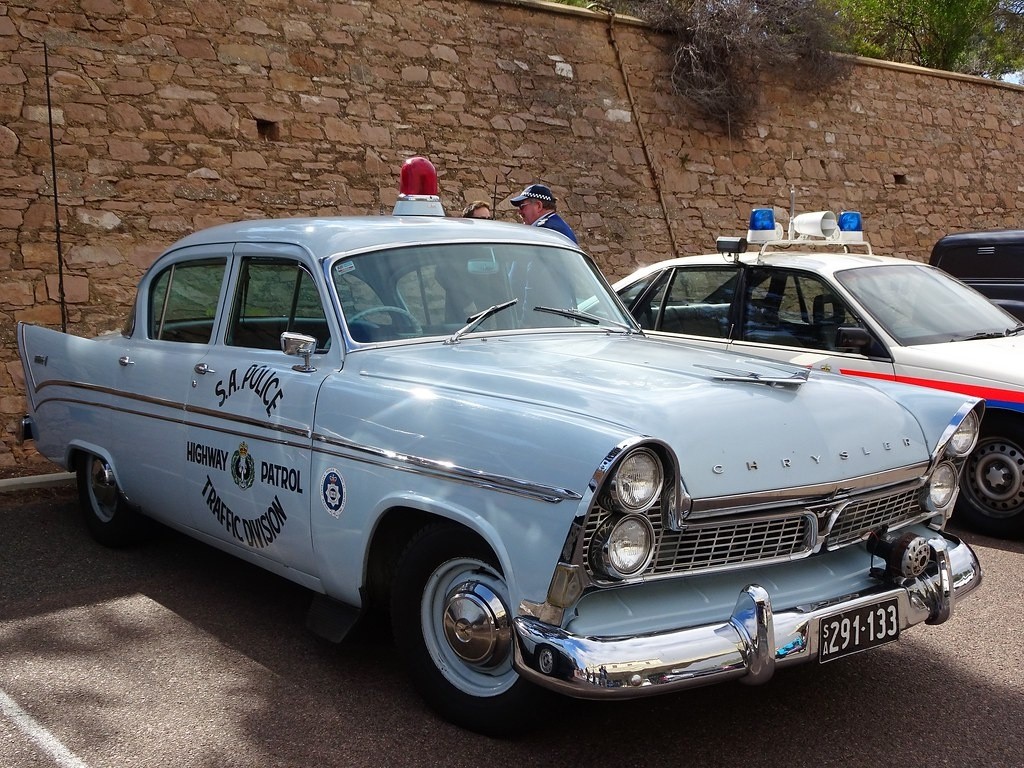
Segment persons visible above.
[511,185,580,330]
[433,200,513,330]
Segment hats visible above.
[510,184,553,207]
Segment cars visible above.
[929,229,1024,323]
[581,187,1024,539]
[17,157,985,736]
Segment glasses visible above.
[518,202,536,210]
